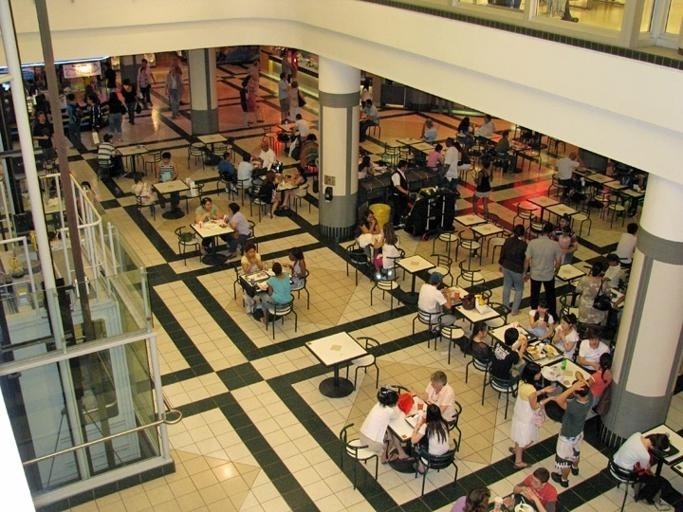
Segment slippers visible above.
[382,453,416,464]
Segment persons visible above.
[238,244,262,278]
[193,197,219,252]
[130,170,157,215]
[609,433,668,505]
[356,80,524,278]
[163,46,320,219]
[259,262,294,328]
[418,272,457,333]
[366,368,461,467]
[283,247,306,293]
[154,148,176,185]
[136,57,155,108]
[223,202,251,261]
[0,58,138,191]
[555,152,587,203]
[452,465,560,512]
[474,213,640,488]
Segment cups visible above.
[454,292,460,301]
[417,403,424,411]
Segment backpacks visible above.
[68,102,83,123]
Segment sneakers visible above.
[551,472,569,487]
[571,465,579,475]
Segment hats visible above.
[104,133,113,141]
[428,271,443,282]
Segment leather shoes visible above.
[509,447,516,455]
[513,462,532,469]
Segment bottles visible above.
[560,356,568,372]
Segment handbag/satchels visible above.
[298,91,305,107]
[120,104,126,115]
[292,146,301,159]
[592,294,610,311]
[135,105,141,114]
[183,233,192,242]
[374,257,382,268]
